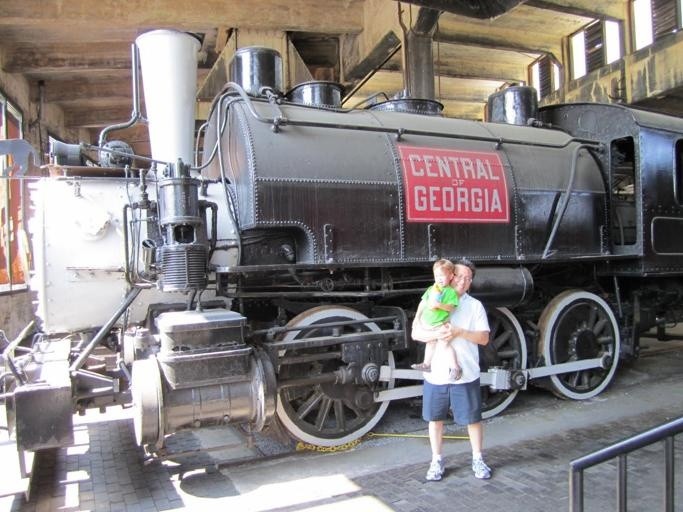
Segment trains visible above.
[0,30,682,479]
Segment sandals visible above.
[410,363,432,372]
[448,367,463,381]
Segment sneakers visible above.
[426,459,446,481]
[472,458,491,479]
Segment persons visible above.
[409,258,462,381]
[409,259,491,484]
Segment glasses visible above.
[454,274,472,283]
